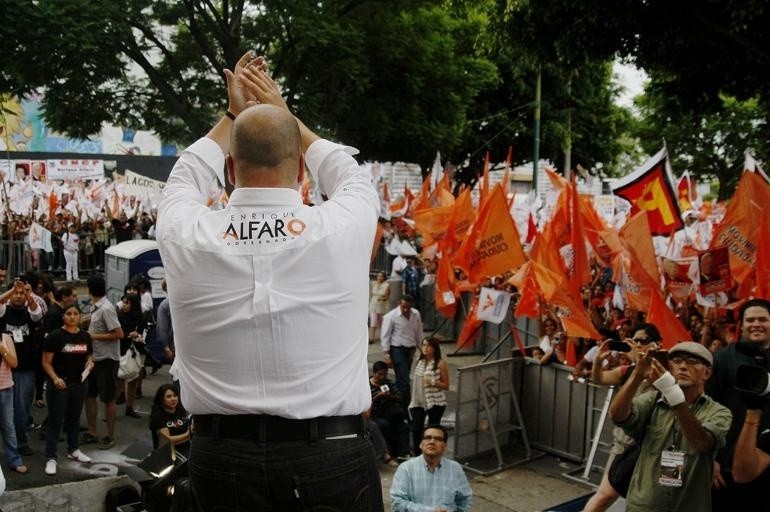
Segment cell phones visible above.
[609,341,631,352]
[19,273,27,284]
[653,351,669,370]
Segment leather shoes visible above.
[116,390,142,418]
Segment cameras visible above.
[553,335,560,343]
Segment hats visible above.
[67,223,75,227]
[667,341,714,367]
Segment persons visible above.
[155,47,382,512]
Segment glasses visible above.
[424,436,444,442]
[632,336,652,345]
[672,357,699,365]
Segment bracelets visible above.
[226,111,237,121]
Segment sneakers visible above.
[14,425,115,474]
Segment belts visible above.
[190,417,362,442]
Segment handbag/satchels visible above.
[608,440,641,499]
[117,345,145,382]
